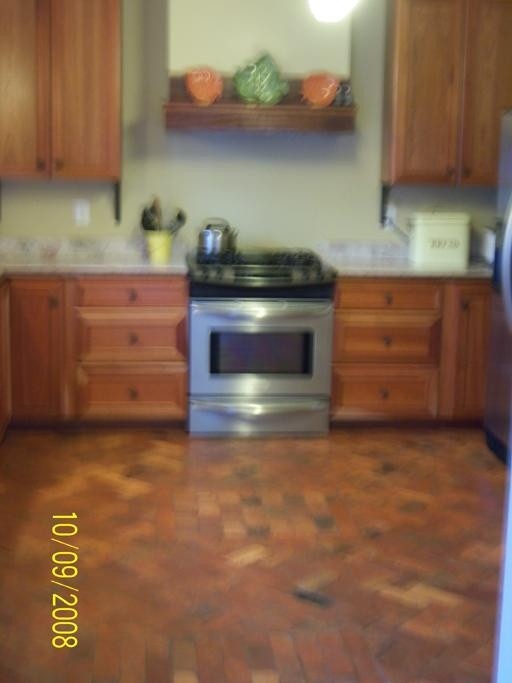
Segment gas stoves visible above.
[185,247,337,289]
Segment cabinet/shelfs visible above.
[445,280,489,422]
[328,278,442,418]
[73,277,189,424]
[7,278,71,422]
[382,0,512,188]
[0,0,124,182]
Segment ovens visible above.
[186,296,335,436]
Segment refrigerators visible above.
[483,104,512,463]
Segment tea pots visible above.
[196,221,240,256]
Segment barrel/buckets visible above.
[142,227,176,267]
